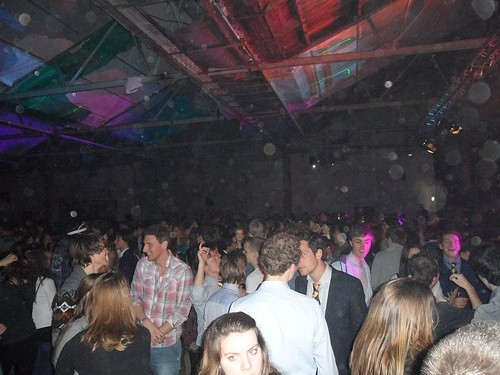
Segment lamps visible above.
[425,141,437,154]
[450,125,462,135]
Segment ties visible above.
[448,261,457,275]
[311,283,321,305]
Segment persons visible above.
[51,232,108,346]
[189,242,223,349]
[0,323,6,334]
[408,252,482,341]
[0,204,500,317]
[129,224,193,375]
[32,252,57,375]
[294,231,367,375]
[419,322,499,375]
[349,277,440,375]
[181,246,199,375]
[228,231,339,375]
[196,312,283,375]
[0,253,48,375]
[51,274,104,368]
[469,243,500,324]
[55,271,152,375]
[196,250,247,347]
